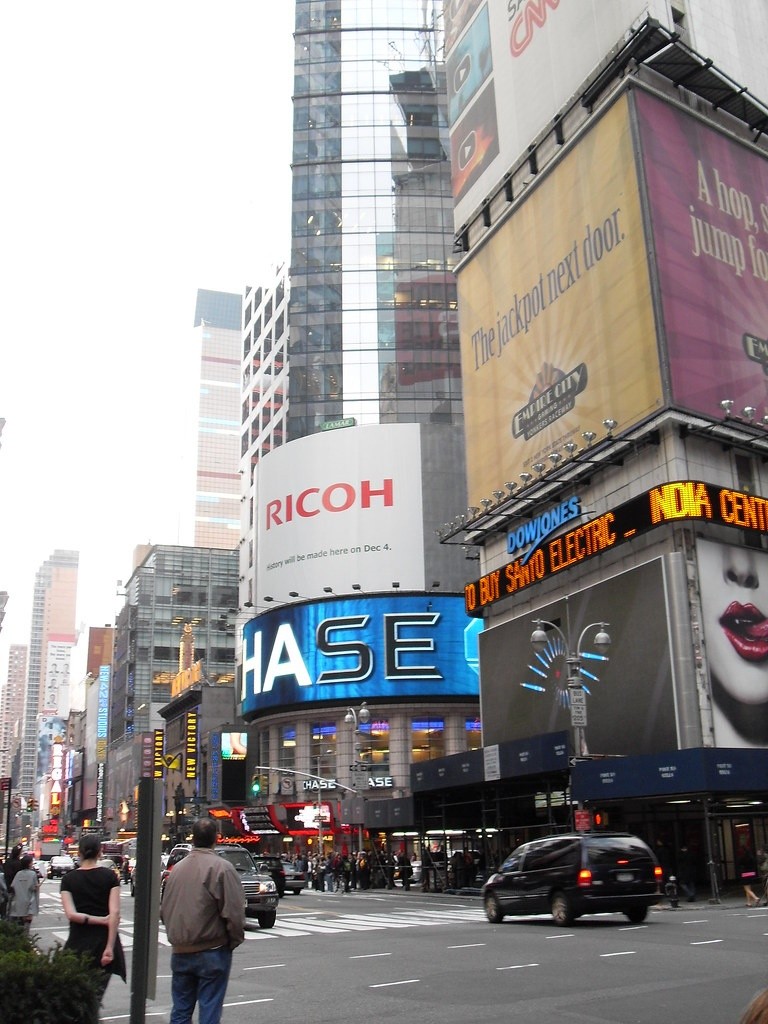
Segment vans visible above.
[482,833,667,928]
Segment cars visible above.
[98,854,134,882]
[257,861,306,896]
[46,856,75,879]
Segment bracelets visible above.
[83,915,89,925]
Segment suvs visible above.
[248,855,286,898]
[161,843,278,928]
[130,852,169,896]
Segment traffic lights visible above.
[27,797,34,812]
[260,775,268,791]
[34,799,39,811]
[252,775,260,792]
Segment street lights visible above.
[530,619,613,830]
[344,702,371,853]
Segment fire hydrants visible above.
[665,877,680,909]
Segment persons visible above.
[259,851,417,893]
[450,846,510,889]
[60,834,126,1011]
[739,986,768,1024]
[0,845,41,934]
[122,856,129,884]
[160,817,245,1024]
[695,537,768,748]
[654,838,706,902]
[740,843,768,907]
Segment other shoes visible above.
[687,893,696,902]
